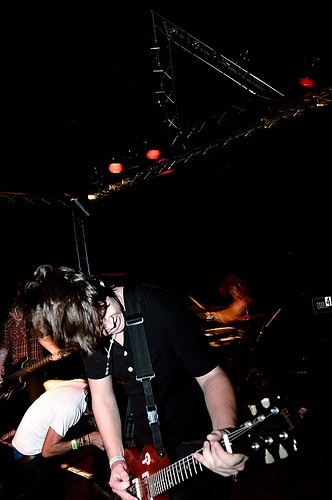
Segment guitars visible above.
[0,341,80,401]
[90,396,300,500]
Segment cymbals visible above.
[190,305,225,312]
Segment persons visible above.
[12,378,105,500]
[211,272,267,324]
[21,266,249,500]
[0,297,63,403]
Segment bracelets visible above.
[109,456,125,469]
[71,439,78,450]
[78,434,91,447]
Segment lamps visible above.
[144,136,169,162]
[297,53,322,90]
[104,151,126,177]
